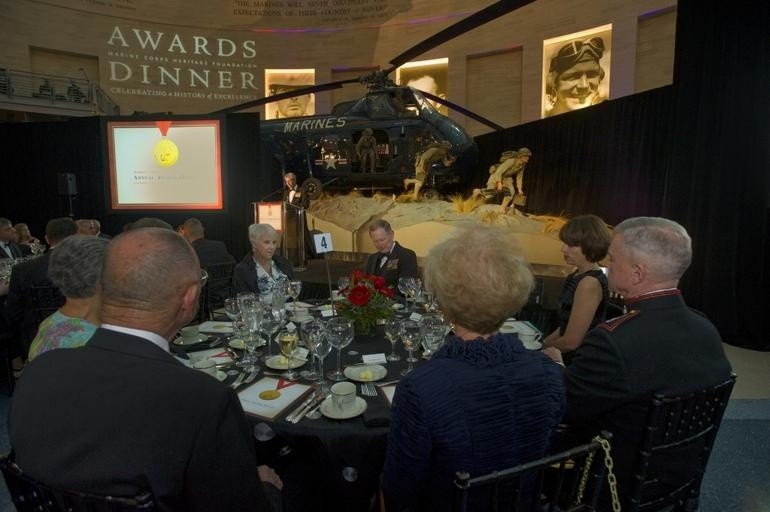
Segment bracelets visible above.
[543,340,551,347]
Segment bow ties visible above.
[377,251,389,261]
[289,188,296,192]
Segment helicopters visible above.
[202,0,539,202]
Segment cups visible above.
[193,360,217,378]
[517,328,541,348]
[330,382,357,412]
[181,326,200,343]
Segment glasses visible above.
[198,270,208,286]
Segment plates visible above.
[174,334,209,346]
[215,369,226,382]
[344,364,388,382]
[320,396,369,420]
[521,341,542,351]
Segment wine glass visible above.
[225,280,354,391]
[0,244,46,284]
[384,278,446,377]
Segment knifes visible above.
[285,391,326,424]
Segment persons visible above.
[66,81,90,102]
[401,67,446,114]
[403,140,459,200]
[0,216,305,512]
[39,80,55,94]
[568,214,733,511]
[363,219,418,294]
[480,147,532,215]
[266,72,315,119]
[539,214,610,353]
[379,227,567,512]
[355,127,379,173]
[284,173,311,209]
[544,37,607,117]
